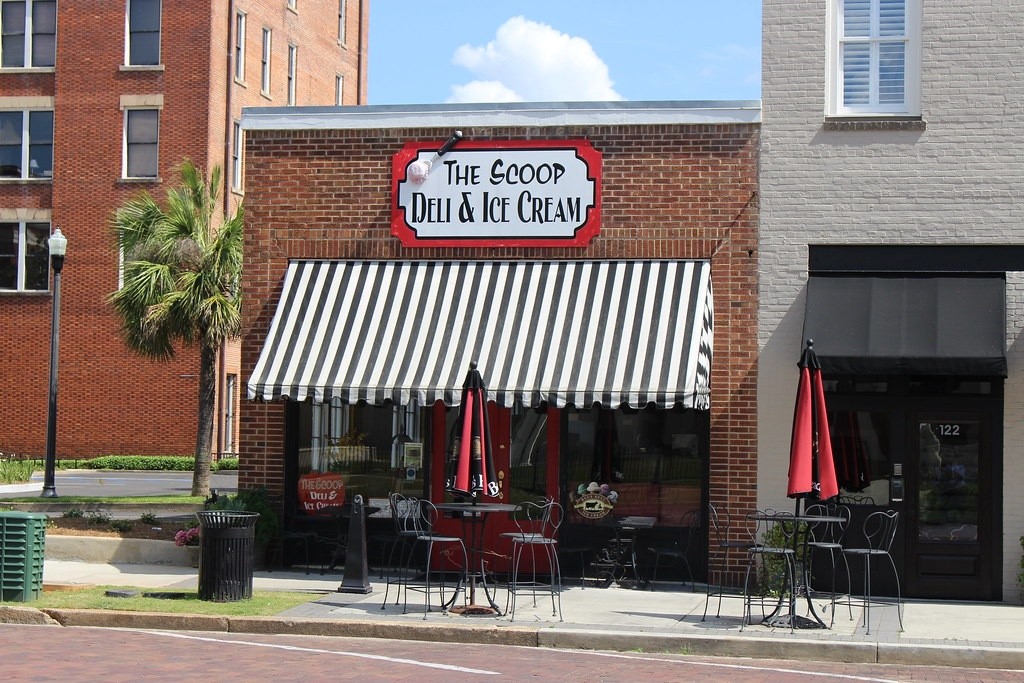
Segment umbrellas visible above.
[832,377,871,492]
[447,361,499,606]
[788,338,838,615]
[591,407,624,485]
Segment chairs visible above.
[285,492,906,639]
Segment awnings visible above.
[246,258,712,410]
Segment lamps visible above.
[29,159,38,176]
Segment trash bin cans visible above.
[195,511,261,603]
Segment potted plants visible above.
[205,485,281,571]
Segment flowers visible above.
[174,527,198,546]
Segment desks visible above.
[746,514,849,629]
[618,513,659,588]
[315,505,380,573]
[425,502,523,618]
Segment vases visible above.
[188,544,198,568]
